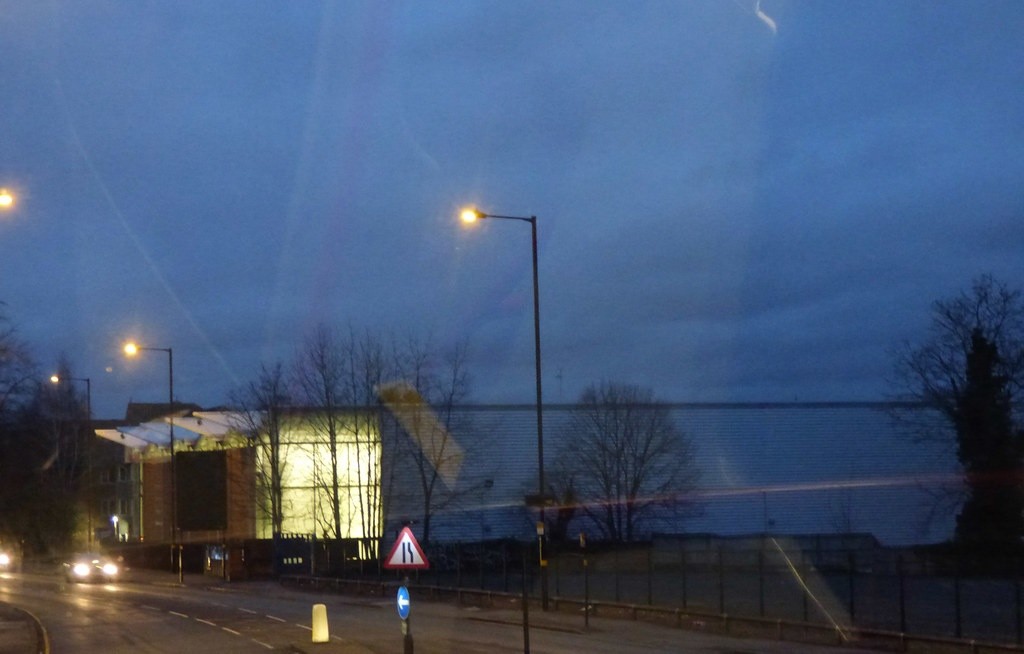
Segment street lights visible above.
[123,342,179,555]
[460,206,552,612]
[112,516,119,545]
[52,374,93,555]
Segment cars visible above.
[63,553,123,583]
[0,537,24,573]
